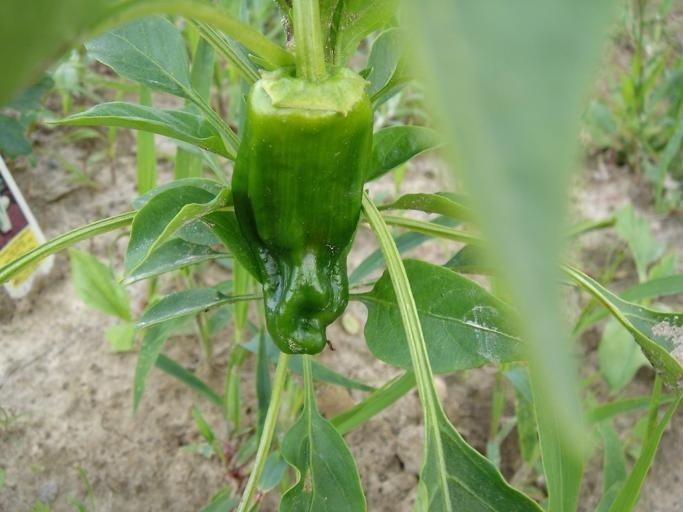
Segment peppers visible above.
[231,79,372,355]
